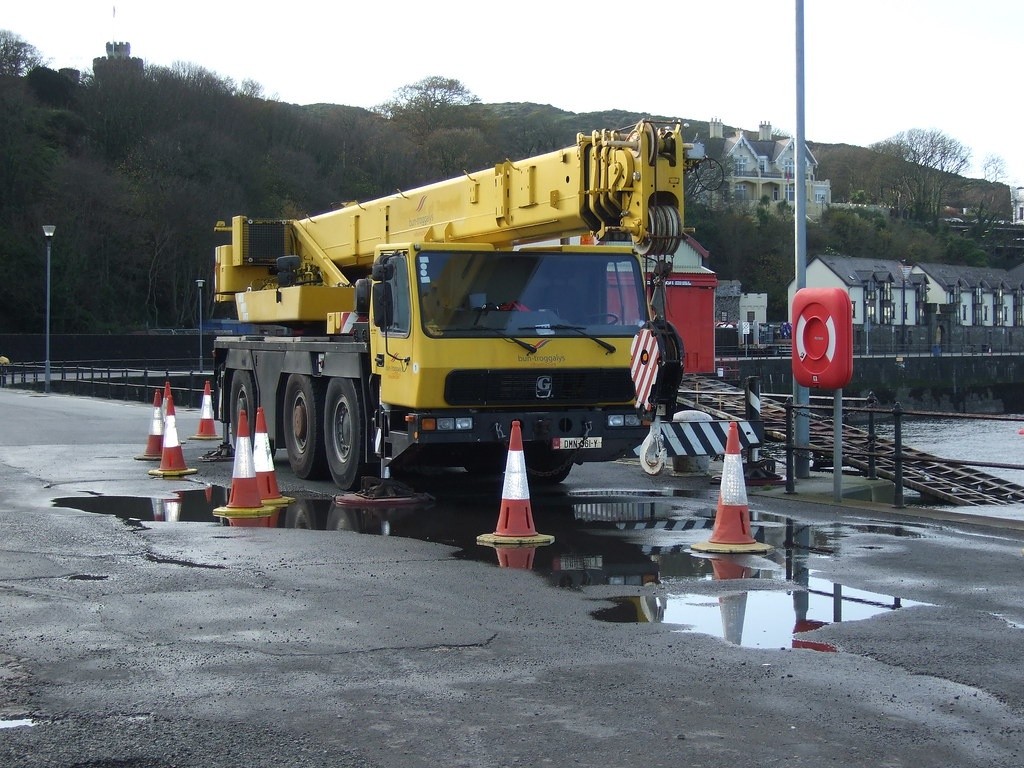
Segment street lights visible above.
[42,223,56,392]
[865,298,869,356]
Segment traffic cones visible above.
[190,380,223,439]
[212,408,295,517]
[691,422,772,554]
[476,420,556,545]
[696,556,755,644]
[135,389,162,458]
[150,382,197,475]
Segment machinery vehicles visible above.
[212,119,695,492]
